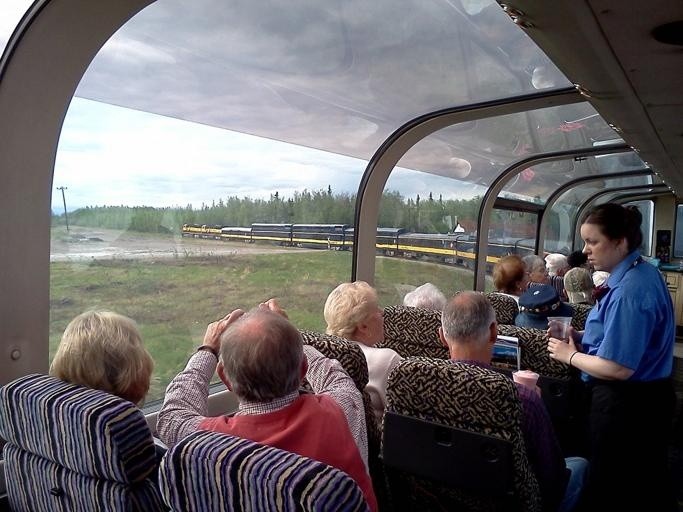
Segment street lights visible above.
[56,186,68,232]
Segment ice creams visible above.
[511,370,539,389]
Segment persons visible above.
[401,250,611,330]
[546,202,675,433]
[48,309,167,462]
[323,280,403,417]
[439,290,567,478]
[156,298,378,510]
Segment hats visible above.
[514,285,572,329]
[564,267,593,305]
[567,251,587,267]
[545,254,566,267]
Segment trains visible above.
[182,221,588,301]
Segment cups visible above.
[547,316,573,346]
[512,368,539,392]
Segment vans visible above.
[59,231,103,244]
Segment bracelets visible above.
[566,351,580,366]
[198,342,218,363]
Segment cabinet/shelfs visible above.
[656,266,683,332]
